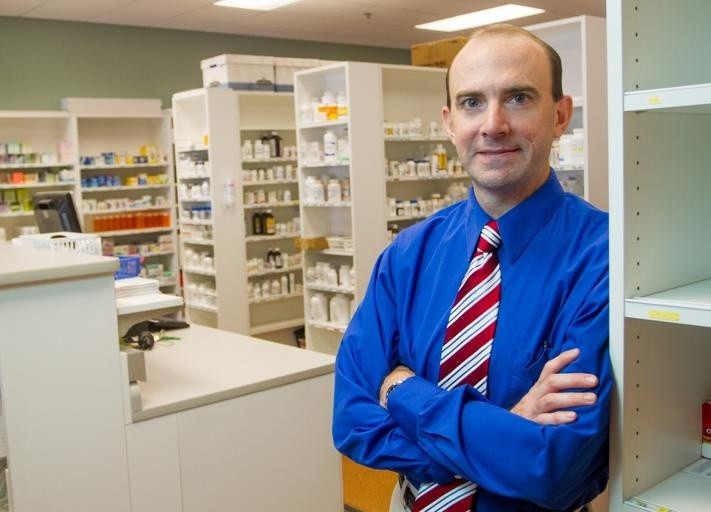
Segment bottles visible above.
[376,113,470,239]
[242,132,304,305]
[300,93,361,325]
[178,156,219,308]
[81,145,174,254]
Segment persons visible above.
[329,21,618,512]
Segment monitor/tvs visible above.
[32,192,82,233]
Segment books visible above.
[114,276,161,301]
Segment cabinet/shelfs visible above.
[0,1,710,512]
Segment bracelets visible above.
[386,383,400,400]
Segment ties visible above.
[411,220,503,512]
